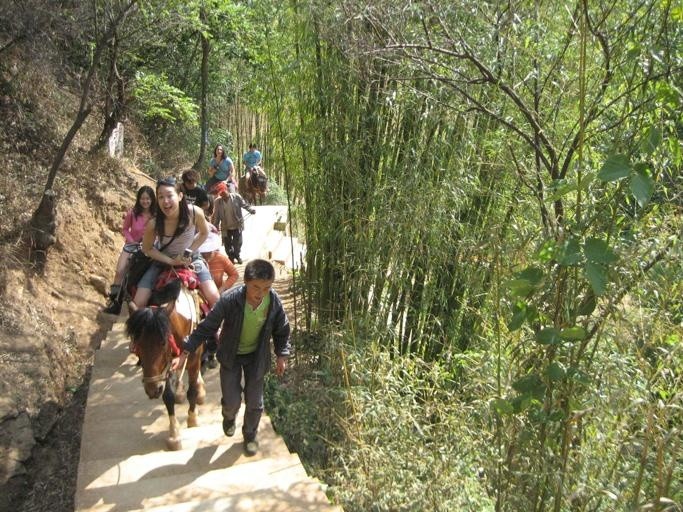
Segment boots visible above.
[104,283,123,316]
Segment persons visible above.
[211,181,256,264]
[242,143,262,188]
[103,176,239,354]
[184,259,290,454]
[206,143,235,194]
[179,168,209,209]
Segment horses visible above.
[238,173,268,205]
[124,276,207,450]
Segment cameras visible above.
[183,248,192,262]
[213,164,219,170]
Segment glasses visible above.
[158,177,177,185]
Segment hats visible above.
[215,183,226,195]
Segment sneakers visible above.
[223,417,236,436]
[243,432,258,455]
[236,256,242,264]
